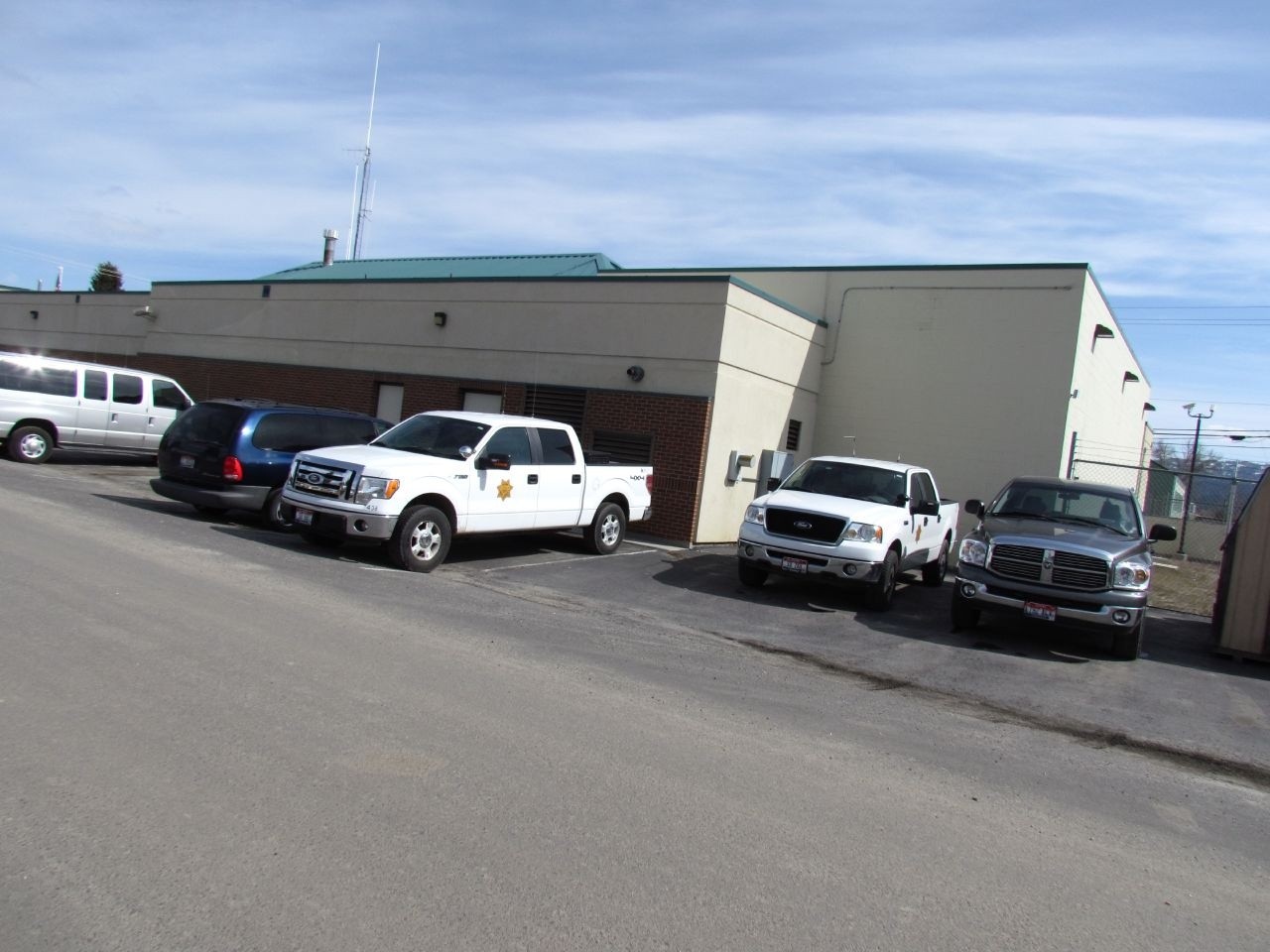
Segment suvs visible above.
[950,474,1178,662]
[148,396,398,522]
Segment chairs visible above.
[1019,496,1041,515]
[880,478,902,503]
[1100,504,1121,521]
[809,469,842,495]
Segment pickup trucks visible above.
[736,457,960,614]
[280,409,654,574]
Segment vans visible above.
[0,350,197,465]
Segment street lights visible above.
[1173,401,1215,560]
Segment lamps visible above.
[1125,371,1139,382]
[30,311,38,318]
[1144,402,1156,411]
[434,312,448,326]
[1095,324,1115,338]
